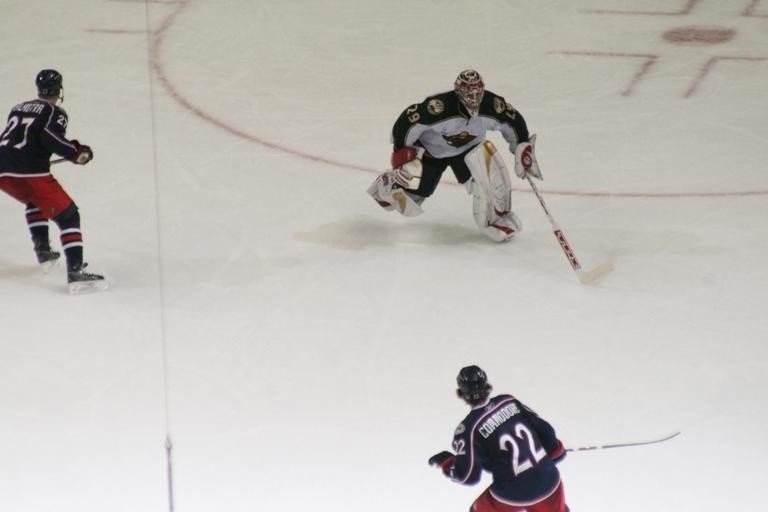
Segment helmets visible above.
[453,70,485,109]
[456,367,493,404]
[35,70,62,96]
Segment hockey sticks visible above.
[524,173,615,286]
[563,430,684,453]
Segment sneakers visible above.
[33,245,60,263]
[68,263,104,283]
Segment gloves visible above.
[64,140,92,165]
[428,451,454,468]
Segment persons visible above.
[0,69,108,283]
[428,366,571,512]
[368,70,543,242]
